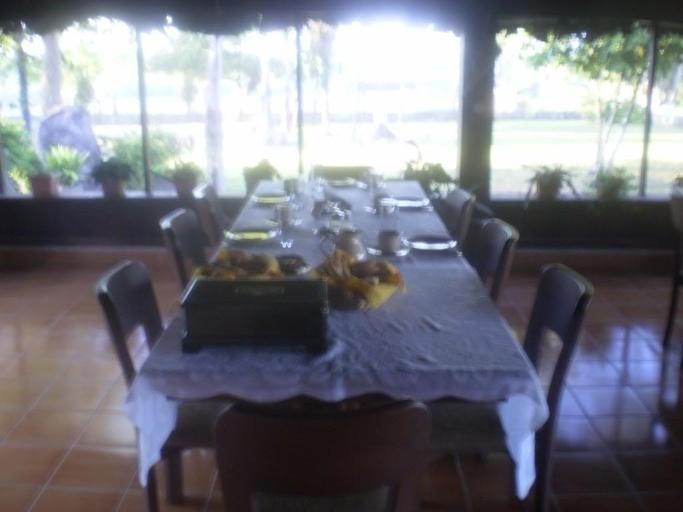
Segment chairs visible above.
[467,217,521,297]
[158,209,209,289]
[661,188,683,350]
[94,259,224,511]
[429,261,594,512]
[437,188,474,248]
[192,181,229,246]
[214,401,432,512]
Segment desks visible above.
[124,179,548,512]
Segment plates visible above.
[222,227,282,243]
[396,195,431,209]
[402,235,458,252]
[250,192,289,205]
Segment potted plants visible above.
[526,166,573,199]
[167,160,208,197]
[8,146,87,197]
[91,157,135,197]
[590,165,634,197]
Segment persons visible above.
[38,70,103,190]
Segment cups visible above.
[377,229,402,253]
